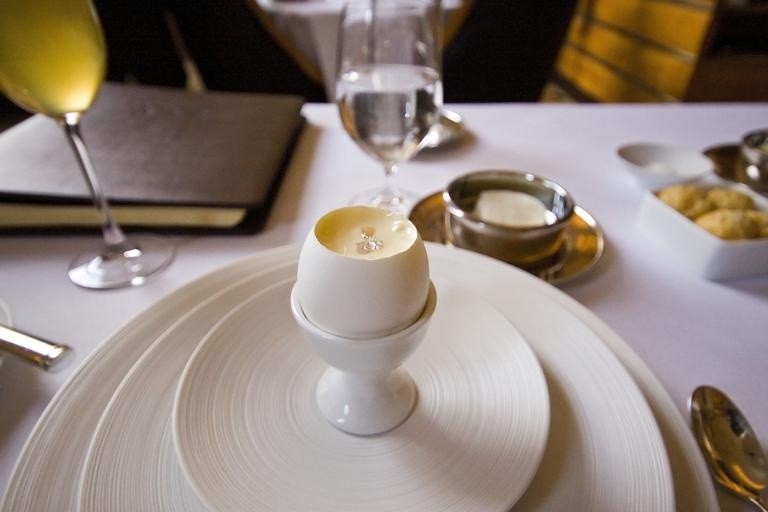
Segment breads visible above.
[656,186,768,245]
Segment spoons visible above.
[690,383,768,512]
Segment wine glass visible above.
[335,0,444,219]
[290,269,438,438]
[0,0,176,290]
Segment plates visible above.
[76,262,677,510]
[6,239,721,512]
[406,187,607,286]
[636,178,767,283]
[170,268,552,512]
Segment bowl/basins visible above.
[613,140,717,194]
[443,169,576,267]
